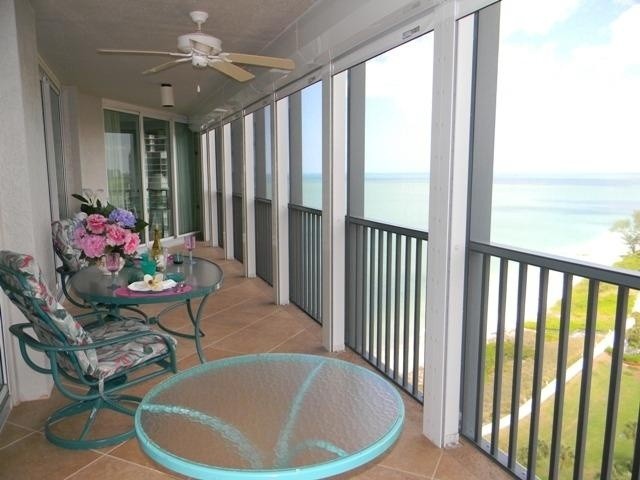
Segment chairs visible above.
[0,251,178,449]
[51,217,143,332]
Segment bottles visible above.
[151,224,164,271]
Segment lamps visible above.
[160,84,175,107]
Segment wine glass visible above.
[105,252,121,289]
[184,235,196,265]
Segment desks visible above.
[134,354,404,480]
[70,255,224,363]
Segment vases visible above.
[96,256,125,275]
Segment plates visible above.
[127,280,152,292]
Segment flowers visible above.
[69,188,148,258]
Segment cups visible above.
[173,250,183,264]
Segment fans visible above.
[96,11,295,82]
